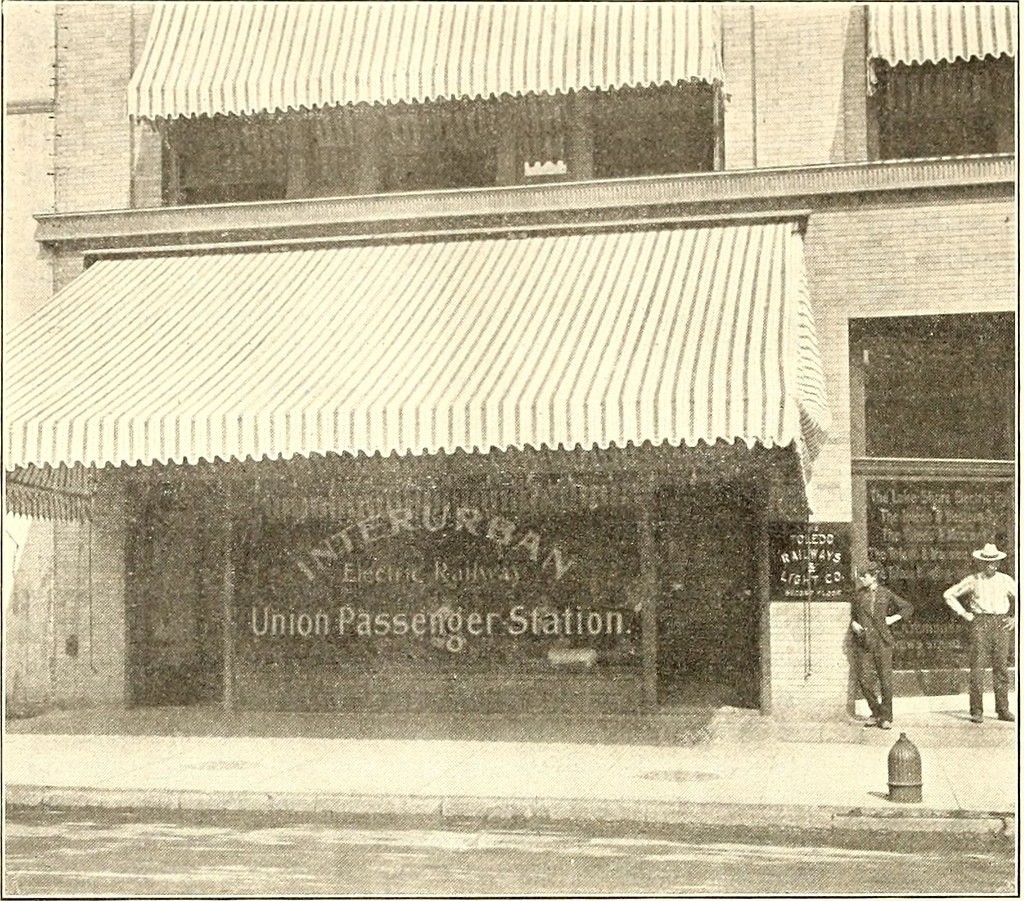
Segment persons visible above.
[847,561,916,733]
[942,544,1016,722]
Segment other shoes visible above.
[864,716,881,726]
[880,720,891,730]
[972,714,983,723]
[999,711,1015,721]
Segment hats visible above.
[972,543,1007,561]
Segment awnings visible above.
[2,209,835,524]
[866,4,1017,98]
[124,2,726,123]
